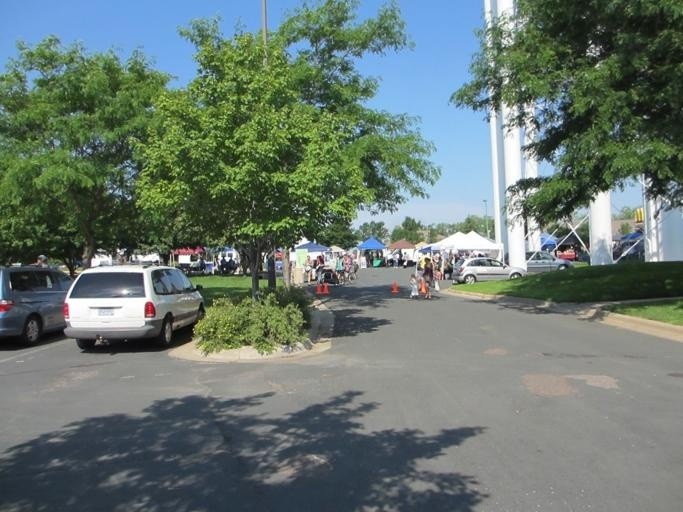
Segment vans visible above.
[63,263,207,351]
[0,263,76,348]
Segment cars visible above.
[499,251,574,273]
[450,256,527,286]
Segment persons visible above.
[198,256,206,272]
[190,257,201,274]
[220,256,227,273]
[228,255,237,275]
[304,253,359,286]
[364,248,487,300]
[37,253,49,269]
